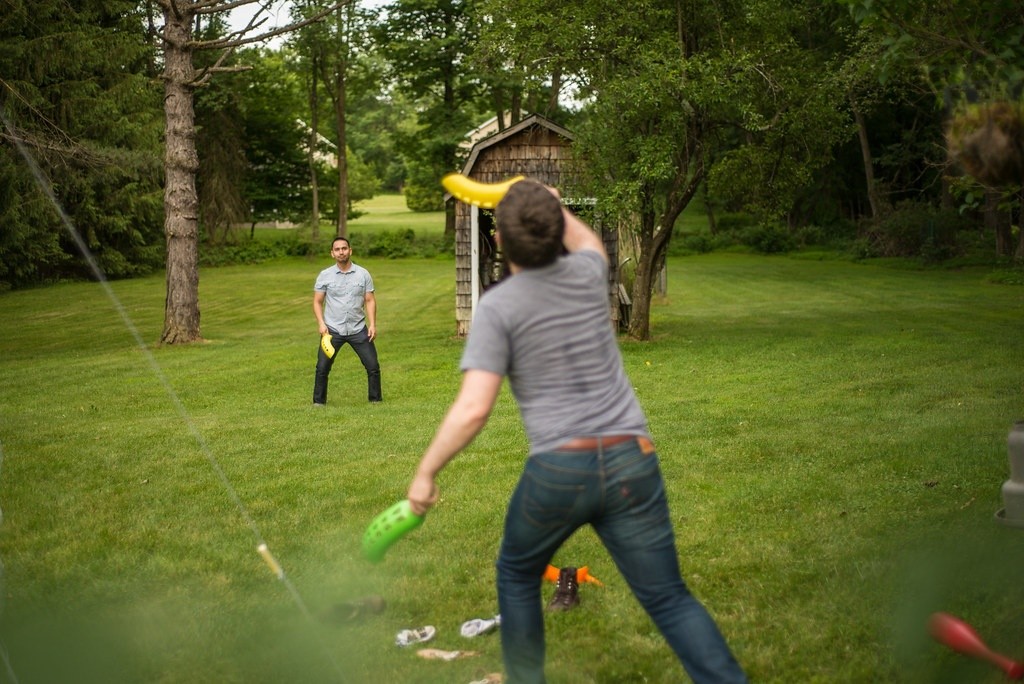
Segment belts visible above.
[560,432,632,451]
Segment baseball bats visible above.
[925,610,1024,680]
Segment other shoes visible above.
[460,613,502,639]
[394,624,437,648]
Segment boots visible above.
[547,566,579,615]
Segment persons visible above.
[313,238,383,404]
[408,180,750,684]
